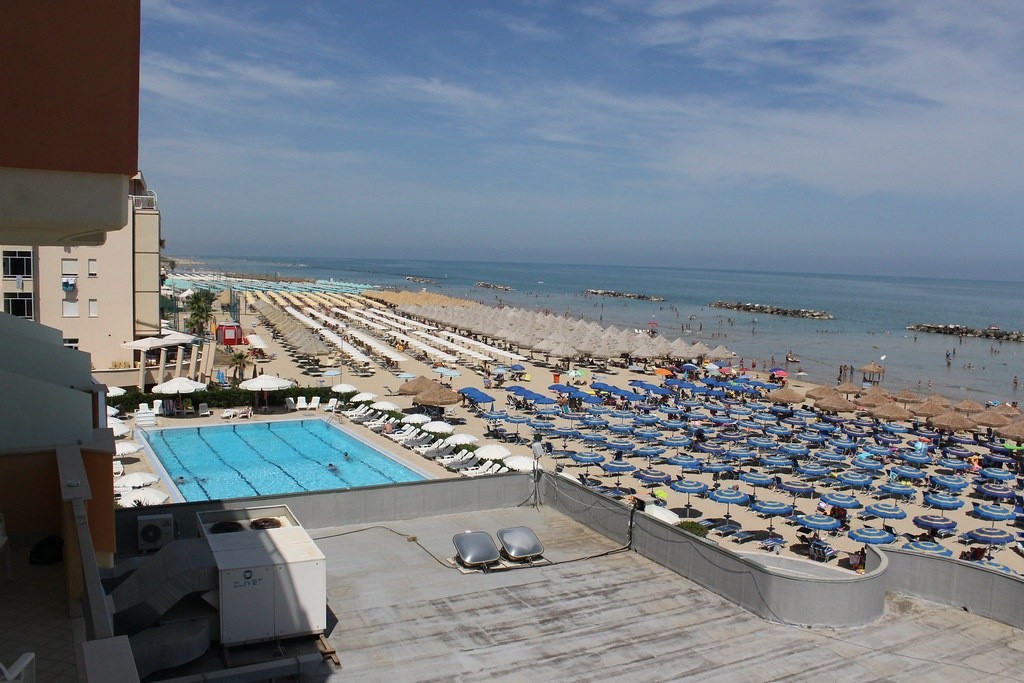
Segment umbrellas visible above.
[399,375,464,419]
[422,363,789,472]
[550,406,1024,574]
[152,376,207,415]
[122,318,269,360]
[285,308,528,376]
[216,368,224,387]
[106,387,169,507]
[401,414,431,440]
[332,383,398,432]
[238,373,294,406]
[250,298,330,371]
[764,383,1024,442]
[161,273,386,308]
[361,290,739,370]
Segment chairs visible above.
[162,271,1024,566]
[112,460,124,477]
[133,400,164,427]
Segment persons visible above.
[174,397,193,410]
[914,335,1018,389]
[657,305,855,378]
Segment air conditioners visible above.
[136,514,175,553]
